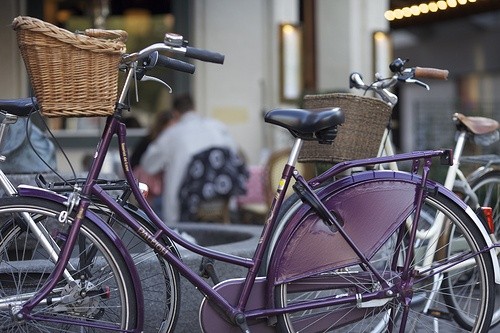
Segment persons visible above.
[131,111,175,196]
[142,94,248,220]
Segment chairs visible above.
[178,146,250,224]
[241,149,318,225]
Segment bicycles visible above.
[0,16,500,332]
[256,58,500,333]
[0,97,184,332]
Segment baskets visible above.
[10,16,128,117]
[297,93,391,169]
